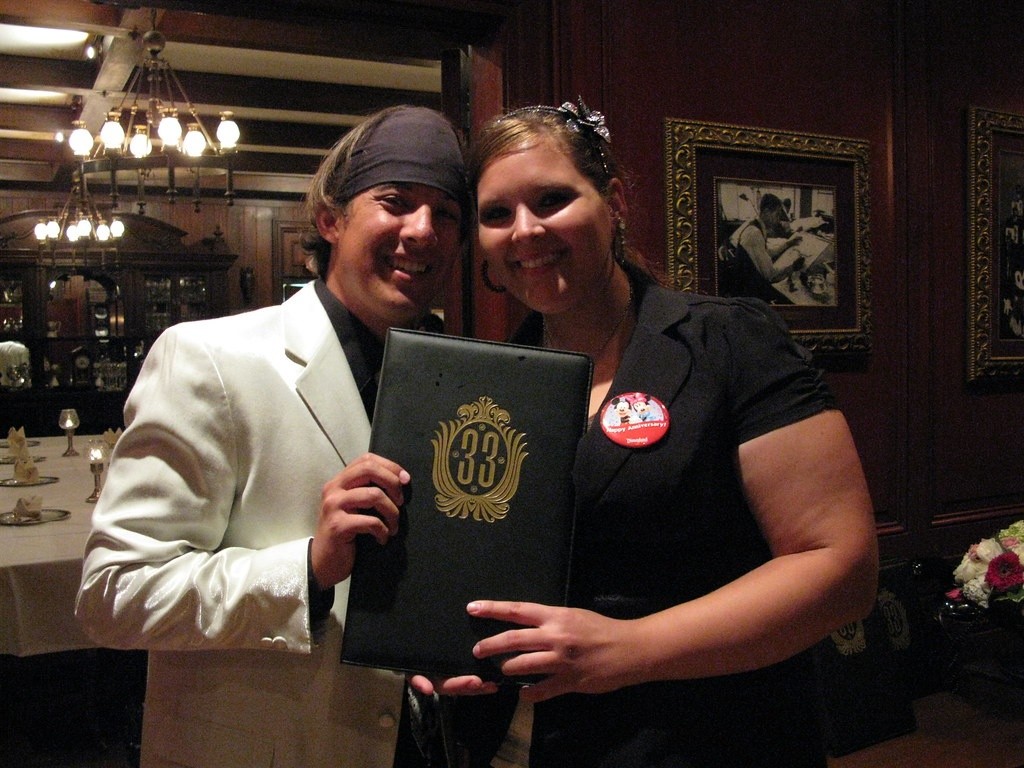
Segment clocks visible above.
[70,345,96,390]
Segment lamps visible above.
[68,0,240,215]
[58,409,81,456]
[32,105,125,273]
[84,437,109,503]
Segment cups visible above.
[47,321,61,337]
[2,318,23,330]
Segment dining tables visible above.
[0,436,124,657]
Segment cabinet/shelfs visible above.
[0,210,240,437]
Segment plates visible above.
[0,440,40,448]
[0,509,71,525]
[0,476,59,486]
[0,456,47,463]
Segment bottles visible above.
[94,307,108,337]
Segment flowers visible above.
[942,519,1024,615]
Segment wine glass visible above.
[59,409,81,457]
[99,360,126,391]
[7,366,21,390]
[18,362,31,387]
[84,438,110,503]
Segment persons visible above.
[720,186,836,305]
[74,105,470,768]
[407,105,879,768]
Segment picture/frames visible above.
[664,116,874,359]
[967,105,1024,385]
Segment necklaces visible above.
[541,292,632,365]
